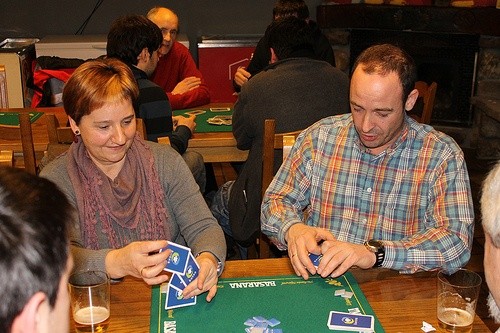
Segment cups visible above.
[435,268,482,333]
[67,269,111,333]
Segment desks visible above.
[69,257,491,333]
[0,107,69,173]
[172,106,249,162]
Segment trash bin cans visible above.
[0,38,39,108]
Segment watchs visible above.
[195,252,222,277]
[364,240,384,268]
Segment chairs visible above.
[409,82,437,125]
[247,119,306,260]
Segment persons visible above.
[99,14,206,193]
[479,161,500,333]
[146,6,219,210]
[211,16,351,261]
[39,58,231,302]
[260,43,474,279]
[233,0,336,94]
[0,165,78,333]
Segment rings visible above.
[291,254,298,258]
[141,267,147,276]
